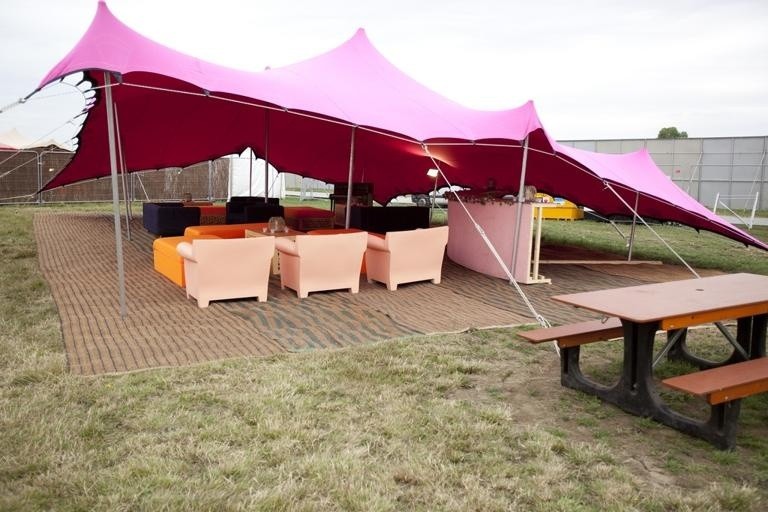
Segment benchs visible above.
[513,317,768,454]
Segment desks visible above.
[444,195,558,286]
[549,270,768,453]
